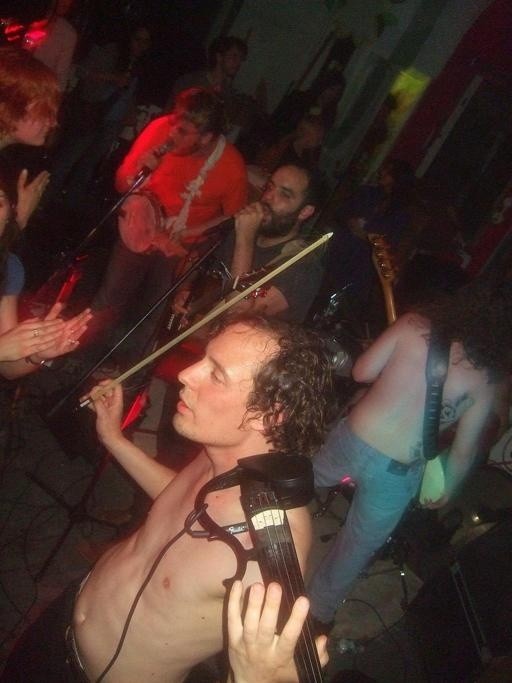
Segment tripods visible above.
[320,505,410,607]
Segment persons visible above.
[1,1,511,683]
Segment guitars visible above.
[368,231,444,507]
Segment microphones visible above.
[329,278,358,300]
[201,217,237,236]
[136,138,174,177]
